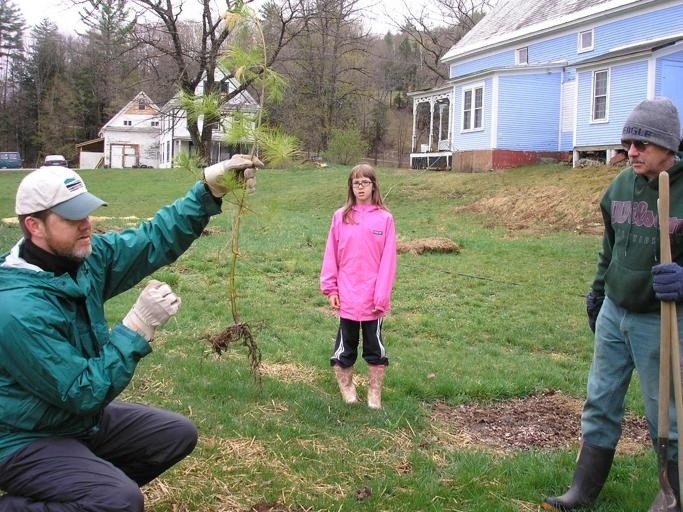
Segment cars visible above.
[43,154,68,169]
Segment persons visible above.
[319,163,396,410]
[542,94,682,511]
[0,153,263,512]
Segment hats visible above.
[621,97,681,153]
[14,166,108,221]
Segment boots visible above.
[540,439,616,511]
[334,364,357,405]
[367,362,385,410]
[642,439,679,511]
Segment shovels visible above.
[646,172,683,512]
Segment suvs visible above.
[0,151,25,170]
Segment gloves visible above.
[651,262,683,303]
[121,279,181,343]
[586,292,605,334]
[202,153,265,197]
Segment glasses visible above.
[621,140,650,153]
[350,180,372,189]
